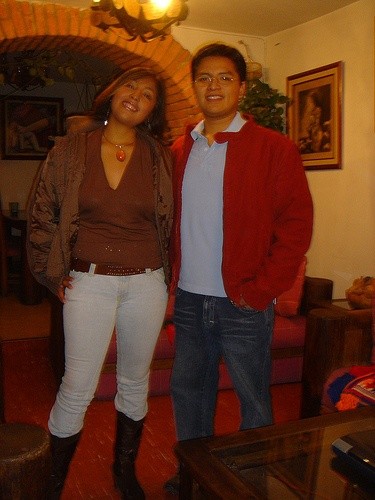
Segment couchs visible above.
[50,276,333,401]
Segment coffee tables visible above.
[172,408,375,500]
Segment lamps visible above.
[91,0,189,43]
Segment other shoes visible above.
[165,476,180,490]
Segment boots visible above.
[47,428,83,500]
[113,410,144,500]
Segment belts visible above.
[70,259,163,277]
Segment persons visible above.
[164,43,314,500]
[27,66,175,500]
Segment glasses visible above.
[192,74,241,87]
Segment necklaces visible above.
[102,131,135,161]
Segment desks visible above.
[3,210,58,305]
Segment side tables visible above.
[297,297,372,419]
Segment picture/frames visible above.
[0,94,64,159]
[284,60,342,172]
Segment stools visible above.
[0,420,51,500]
